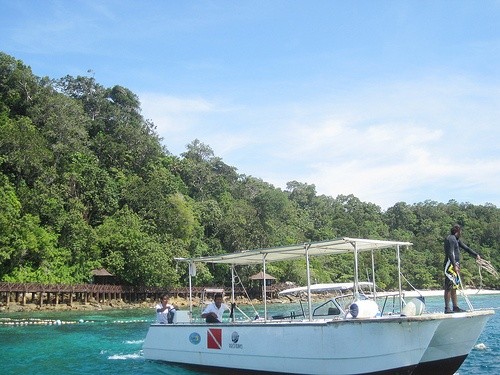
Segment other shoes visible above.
[453,306,466,312]
[445,307,454,314]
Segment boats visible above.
[142,236,496,375]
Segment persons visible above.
[155,293,176,325]
[201,293,230,324]
[442,225,481,315]
